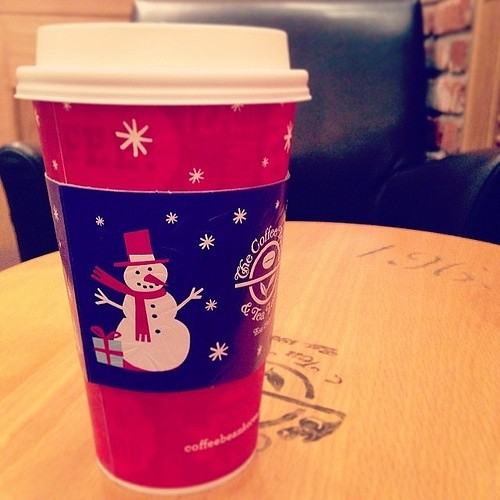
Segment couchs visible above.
[0,0,500,263]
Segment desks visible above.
[0,219,500,500]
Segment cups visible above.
[15,22,312,495]
